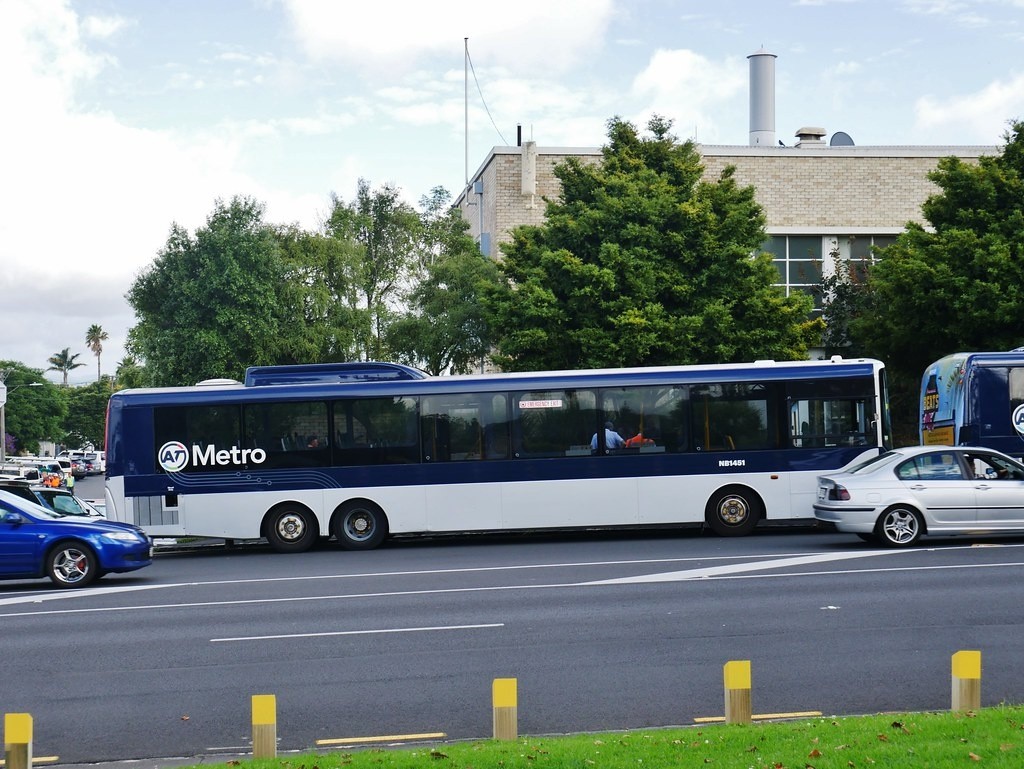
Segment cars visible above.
[811,444,1023,546]
[0,442,105,516]
[0,492,153,587]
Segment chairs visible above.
[159,421,858,456]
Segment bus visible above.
[104,354,887,549]
[919,346,1023,463]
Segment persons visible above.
[626,432,655,448]
[965,456,975,476]
[590,421,626,451]
[65,471,76,496]
[41,472,60,488]
[308,436,319,448]
